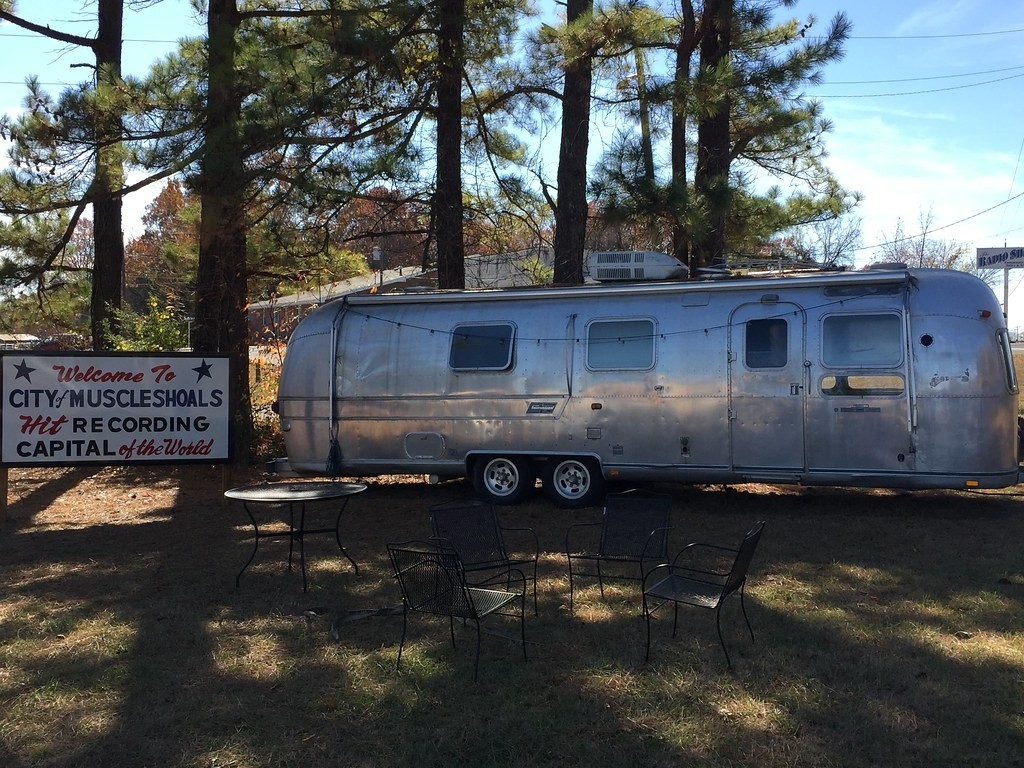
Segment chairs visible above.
[644,519,767,671]
[564,488,673,622]
[427,496,539,630]
[385,539,528,687]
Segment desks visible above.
[223,481,369,595]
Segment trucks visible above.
[271,249,1022,506]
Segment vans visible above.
[36,333,82,351]
